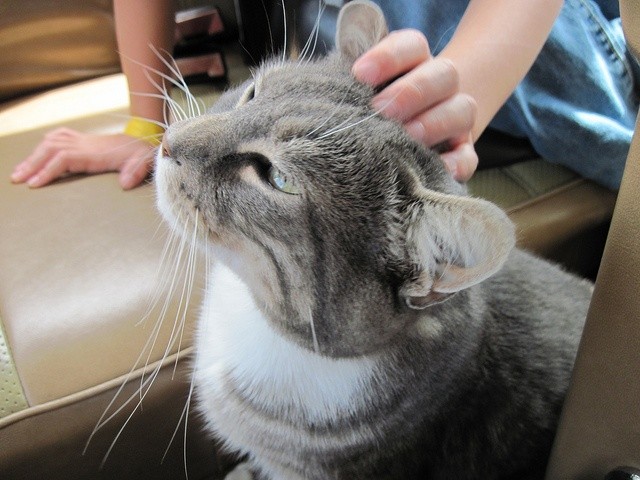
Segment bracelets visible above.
[121,120,165,146]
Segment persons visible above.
[9,0,637,189]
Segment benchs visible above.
[0,0,616,480]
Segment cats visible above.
[83,0,640,480]
